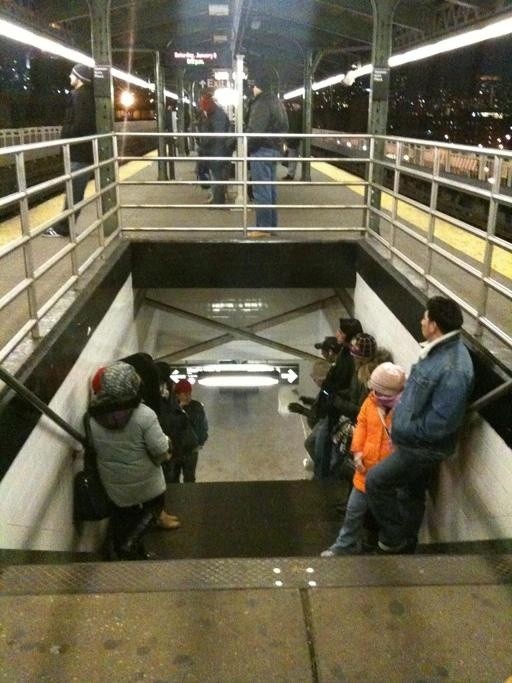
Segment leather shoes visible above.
[157,510,180,530]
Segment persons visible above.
[167,64,303,236]
[85,350,209,561]
[367,296,476,556]
[288,320,394,533]
[322,362,404,556]
[43,63,92,238]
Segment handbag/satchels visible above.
[74,469,112,522]
[177,425,198,453]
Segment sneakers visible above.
[42,228,62,237]
[320,549,335,558]
[246,228,270,239]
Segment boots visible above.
[117,509,156,560]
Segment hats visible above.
[254,76,270,91]
[314,337,343,354]
[366,361,406,396]
[92,360,145,404]
[200,95,214,112]
[173,378,192,393]
[348,333,377,360]
[72,63,92,83]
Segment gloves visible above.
[288,396,315,413]
[354,452,367,473]
